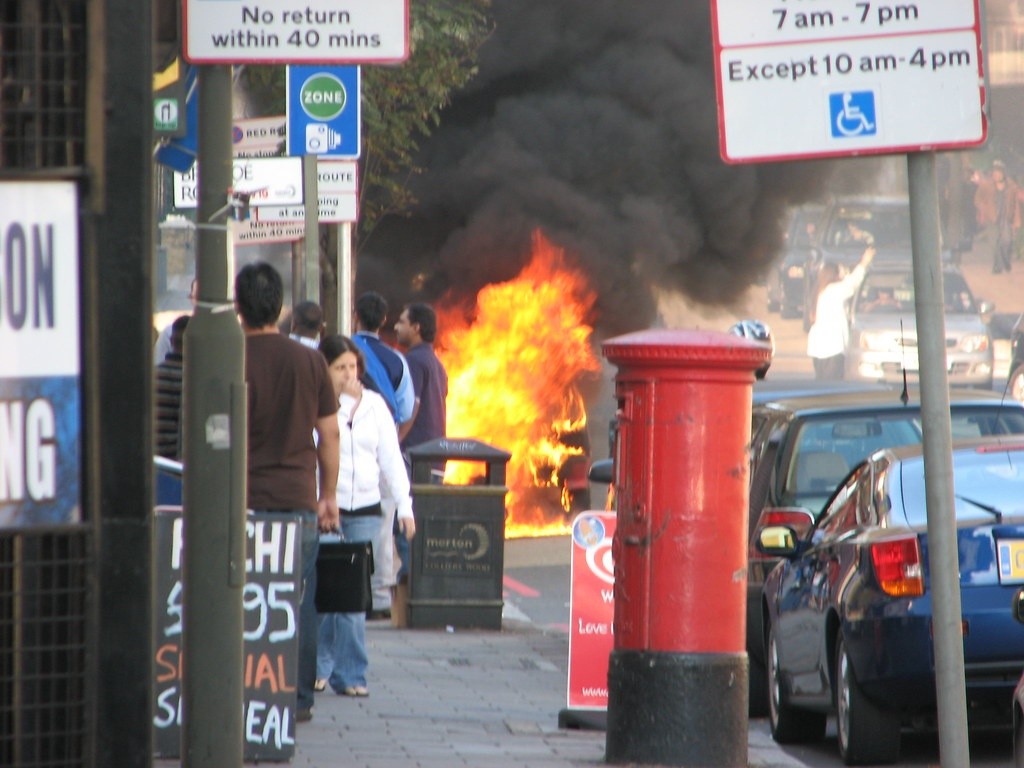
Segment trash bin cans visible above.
[389,438,511,630]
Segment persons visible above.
[934,150,1024,273]
[729,319,773,381]
[234,260,343,720]
[150,275,450,698]
[805,242,877,379]
[978,302,1024,378]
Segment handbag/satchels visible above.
[314,528,373,615]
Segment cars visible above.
[758,434,1023,768]
[845,271,997,390]
[763,204,834,320]
[747,378,1024,721]
[800,195,914,331]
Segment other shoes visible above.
[315,678,326,693]
[346,685,369,698]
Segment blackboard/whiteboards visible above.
[153,509,301,762]
